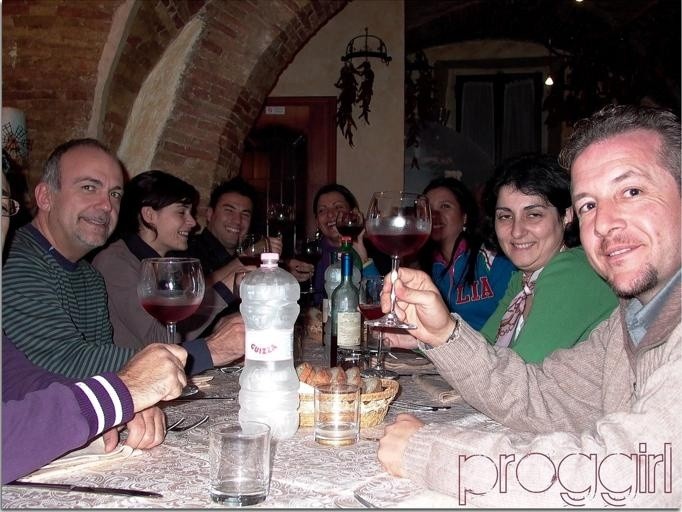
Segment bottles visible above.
[240,252,320,440]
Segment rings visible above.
[296,265,299,272]
[243,272,246,277]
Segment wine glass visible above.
[135,255,206,399]
[323,187,435,382]
[297,232,322,295]
[271,203,295,238]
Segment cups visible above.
[313,384,363,446]
[238,234,270,267]
[205,420,272,508]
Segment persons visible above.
[1,102,681,509]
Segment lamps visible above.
[543,43,573,87]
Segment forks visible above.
[12,478,165,499]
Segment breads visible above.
[294,361,383,397]
